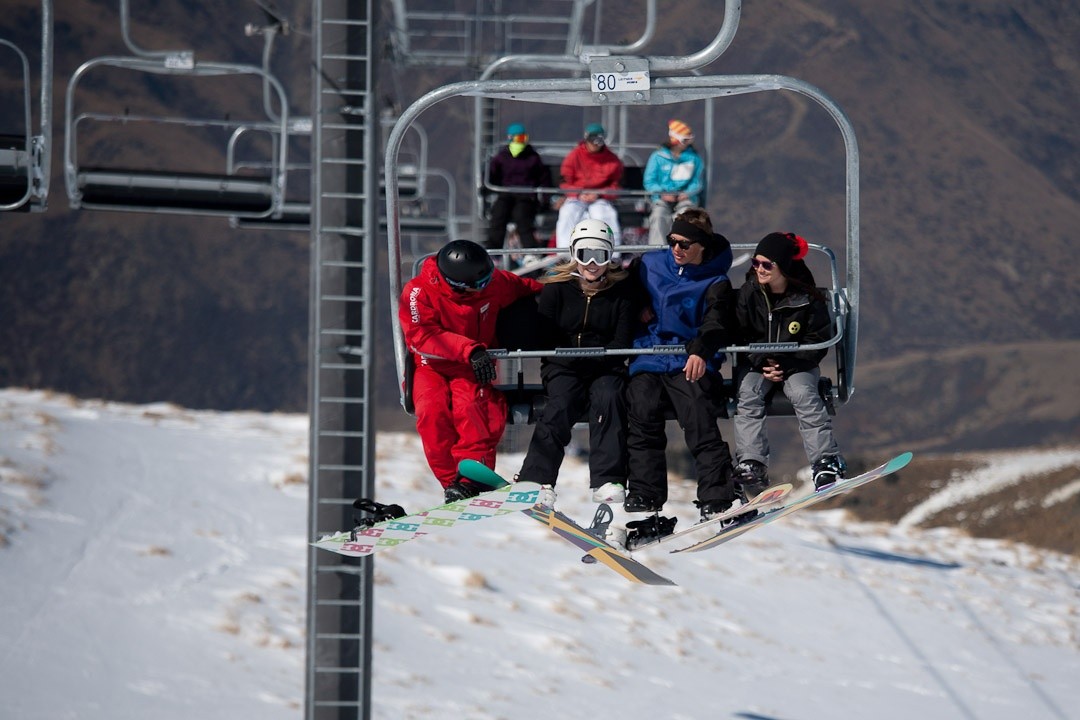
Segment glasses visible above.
[512,134,526,145]
[468,275,493,289]
[679,134,695,145]
[574,247,612,267]
[666,232,695,250]
[751,256,775,270]
[588,135,605,146]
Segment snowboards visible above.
[307,481,546,557]
[629,483,793,552]
[671,452,913,553]
[457,458,678,586]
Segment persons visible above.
[398,240,544,503]
[514,219,637,504]
[551,122,633,264]
[732,231,848,498]
[477,121,556,266]
[623,208,733,518]
[643,119,707,254]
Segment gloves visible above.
[470,346,496,387]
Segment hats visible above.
[506,122,527,145]
[583,123,605,148]
[668,119,692,145]
[754,232,799,264]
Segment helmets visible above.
[568,219,615,266]
[437,240,495,291]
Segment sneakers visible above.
[624,489,665,512]
[591,482,624,505]
[701,477,749,521]
[733,461,772,496]
[811,456,848,488]
[533,483,558,508]
[444,483,479,502]
